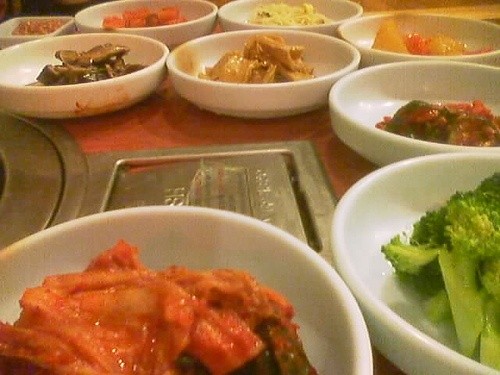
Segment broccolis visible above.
[382,173,500,373]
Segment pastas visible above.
[217,0,362,29]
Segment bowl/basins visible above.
[72,0,218,49]
[328,59,500,166]
[328,150,500,374]
[0,203,375,375]
[217,1,364,38]
[0,16,74,50]
[1,32,171,121]
[166,29,361,120]
[336,10,500,68]
